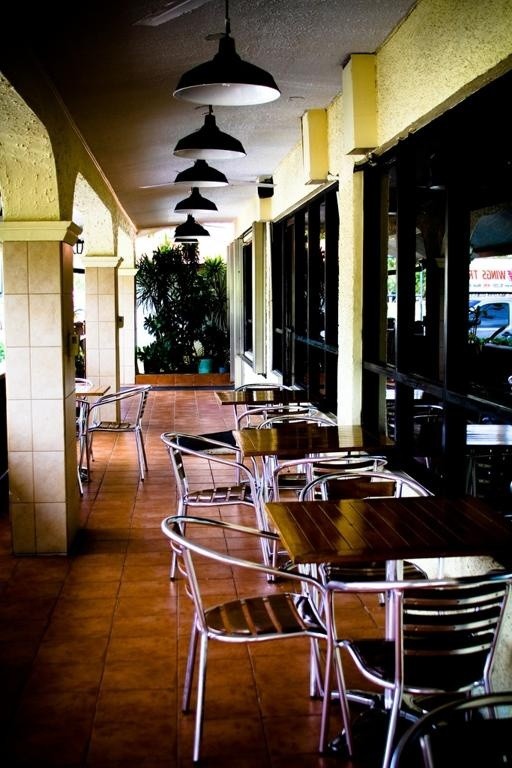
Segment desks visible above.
[261,496,508,748]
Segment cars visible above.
[469,299,512,335]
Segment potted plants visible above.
[133,234,230,374]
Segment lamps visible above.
[74,239,85,255]
[174,159,229,244]
[172,106,247,159]
[173,1,282,108]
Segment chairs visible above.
[85,383,152,482]
[300,474,432,644]
[387,685,511,768]
[159,509,330,762]
[324,568,512,764]
[75,377,91,383]
[75,398,90,482]
[162,384,396,579]
